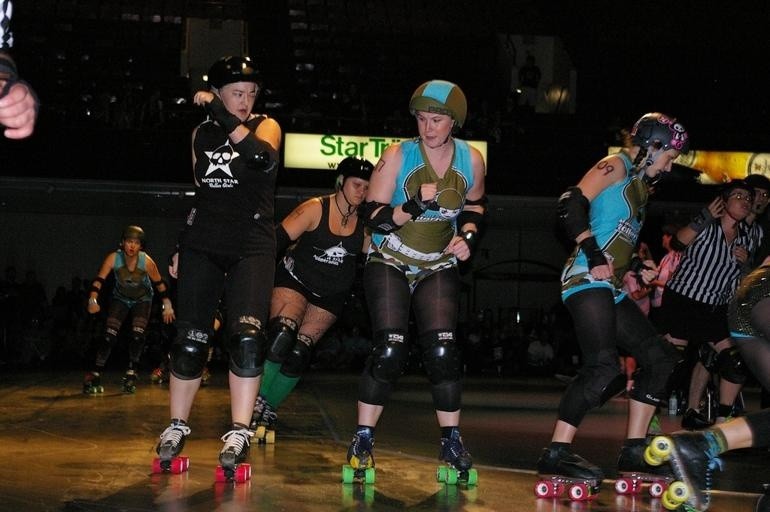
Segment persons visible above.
[347,78,491,472]
[243,155,379,433]
[49,45,285,127]
[455,297,579,379]
[80,225,178,390]
[666,257,770,510]
[146,239,211,383]
[0,0,41,141]
[534,111,691,484]
[619,226,689,397]
[735,173,770,415]
[660,177,754,434]
[154,53,283,469]
[310,320,376,375]
[0,265,108,371]
[291,21,544,149]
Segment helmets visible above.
[338,155,374,183]
[721,174,753,205]
[406,81,471,132]
[120,226,147,244]
[207,55,269,93]
[746,176,769,191]
[627,103,691,155]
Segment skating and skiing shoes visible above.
[147,367,168,384]
[341,427,383,489]
[151,420,193,474]
[249,396,267,440]
[82,371,105,395]
[652,430,717,511]
[202,367,213,387]
[532,441,607,501]
[121,370,138,393]
[255,405,283,445]
[436,428,481,488]
[612,443,678,499]
[212,427,252,485]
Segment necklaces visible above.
[332,192,359,229]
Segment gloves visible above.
[636,262,659,285]
[731,247,757,268]
[166,244,179,278]
[579,237,613,282]
[199,97,243,135]
[445,231,479,260]
[689,205,718,231]
[403,183,434,222]
[1,60,41,144]
